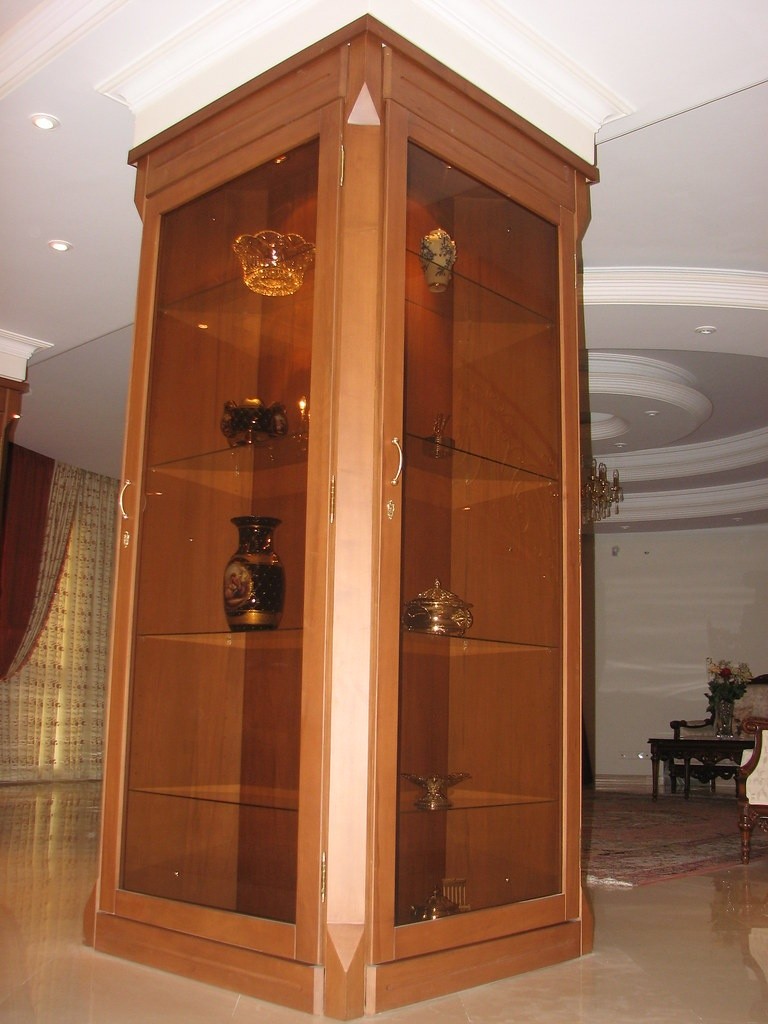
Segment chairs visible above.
[733,717,768,865]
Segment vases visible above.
[712,700,733,738]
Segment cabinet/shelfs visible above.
[84,14,600,1020]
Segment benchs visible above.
[670,674,768,794]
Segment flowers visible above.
[703,660,754,735]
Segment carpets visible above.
[580,793,768,887]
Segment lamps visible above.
[574,422,624,525]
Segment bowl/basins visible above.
[234,231,314,297]
[221,399,287,444]
